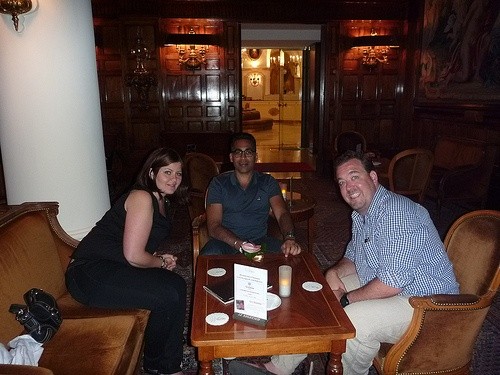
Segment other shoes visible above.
[229,360,276,375]
[142,363,191,375]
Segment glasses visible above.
[230,148,257,157]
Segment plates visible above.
[266,292,281,311]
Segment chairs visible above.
[192,212,208,277]
[0,201,150,375]
[377,148,435,208]
[369,210,500,375]
[181,152,221,278]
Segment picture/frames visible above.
[412,0,500,112]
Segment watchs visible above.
[340,293,349,307]
[288,232,294,238]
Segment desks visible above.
[189,250,358,375]
[270,192,316,255]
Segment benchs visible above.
[242,109,274,131]
[430,135,488,212]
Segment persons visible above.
[199,132,302,258]
[65,147,188,375]
[229,153,462,375]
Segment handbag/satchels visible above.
[9,288,63,346]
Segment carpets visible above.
[255,162,314,172]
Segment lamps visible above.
[126,26,156,112]
[0,0,32,32]
[360,29,392,68]
[176,26,208,74]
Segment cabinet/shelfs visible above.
[241,100,301,121]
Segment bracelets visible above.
[233,240,238,246]
[156,255,165,268]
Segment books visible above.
[203,278,273,304]
